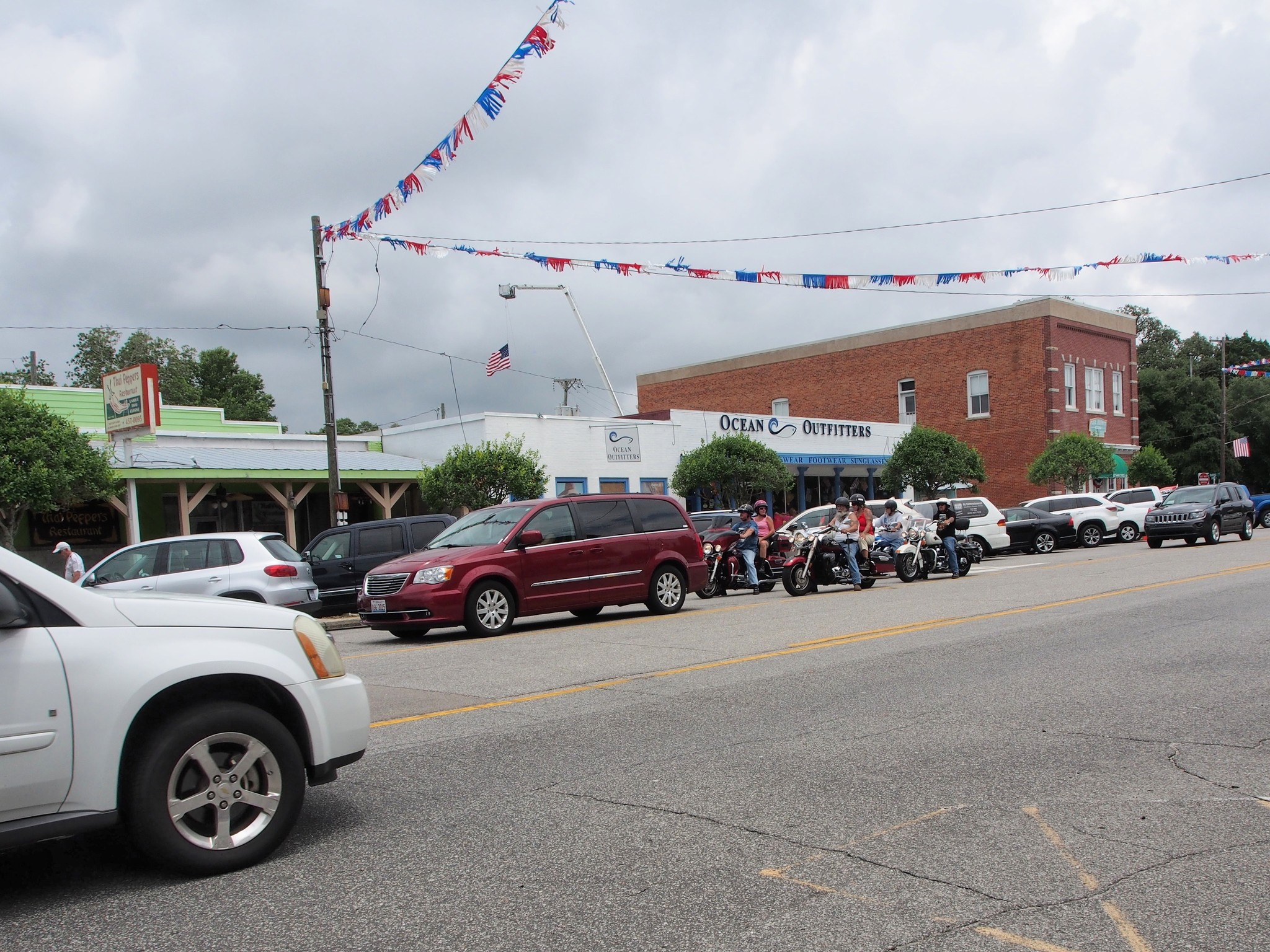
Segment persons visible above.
[916,497,960,579]
[752,500,775,572]
[849,494,875,569]
[872,500,904,564]
[820,497,862,592]
[730,504,760,595]
[775,507,796,522]
[52,542,86,583]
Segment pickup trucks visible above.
[1106,486,1185,508]
[1239,485,1270,528]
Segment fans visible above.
[200,485,253,502]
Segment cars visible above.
[0,546,371,880]
[984,507,1077,557]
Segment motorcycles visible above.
[782,522,897,597]
[695,515,779,599]
[894,514,981,583]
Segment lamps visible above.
[210,498,229,509]
[350,489,372,500]
[538,414,547,421]
[357,498,372,505]
[190,456,198,464]
[56,511,65,522]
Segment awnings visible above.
[1094,453,1129,478]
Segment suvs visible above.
[357,491,710,641]
[683,509,759,534]
[73,531,323,618]
[1144,482,1256,549]
[1007,492,1120,549]
[775,496,926,532]
[909,496,1011,560]
[300,513,460,618]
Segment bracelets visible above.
[895,526,898,529]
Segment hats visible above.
[52,541,70,553]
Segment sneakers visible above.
[753,588,759,594]
[854,584,861,591]
[713,589,727,596]
[808,587,818,592]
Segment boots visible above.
[758,558,766,573]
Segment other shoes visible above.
[951,573,959,579]
[915,574,928,580]
[858,557,870,569]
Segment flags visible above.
[1232,437,1251,457]
[486,343,511,377]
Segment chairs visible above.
[185,548,203,570]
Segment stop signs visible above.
[1199,473,1209,485]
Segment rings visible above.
[844,530,846,532]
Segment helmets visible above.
[738,504,754,515]
[849,494,865,503]
[936,497,950,508]
[835,497,849,505]
[754,500,768,510]
[884,500,897,508]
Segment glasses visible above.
[852,503,857,506]
[937,503,945,507]
[740,511,746,513]
[58,548,66,554]
[759,507,766,510]
